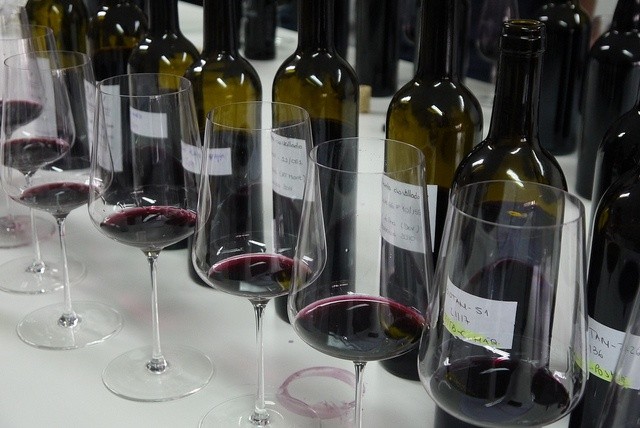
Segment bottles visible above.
[575,0,639,200]
[85,1,160,206]
[379,0,485,381]
[127,0,203,250]
[436,19,567,427]
[568,172,639,427]
[536,1,591,155]
[272,1,360,325]
[180,0,264,289]
[353,2,400,99]
[19,1,98,170]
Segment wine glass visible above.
[0,4,56,249]
[191,100,328,428]
[417,179,588,427]
[286,136,434,427]
[2,49,124,351]
[86,71,216,404]
[3,24,86,294]
